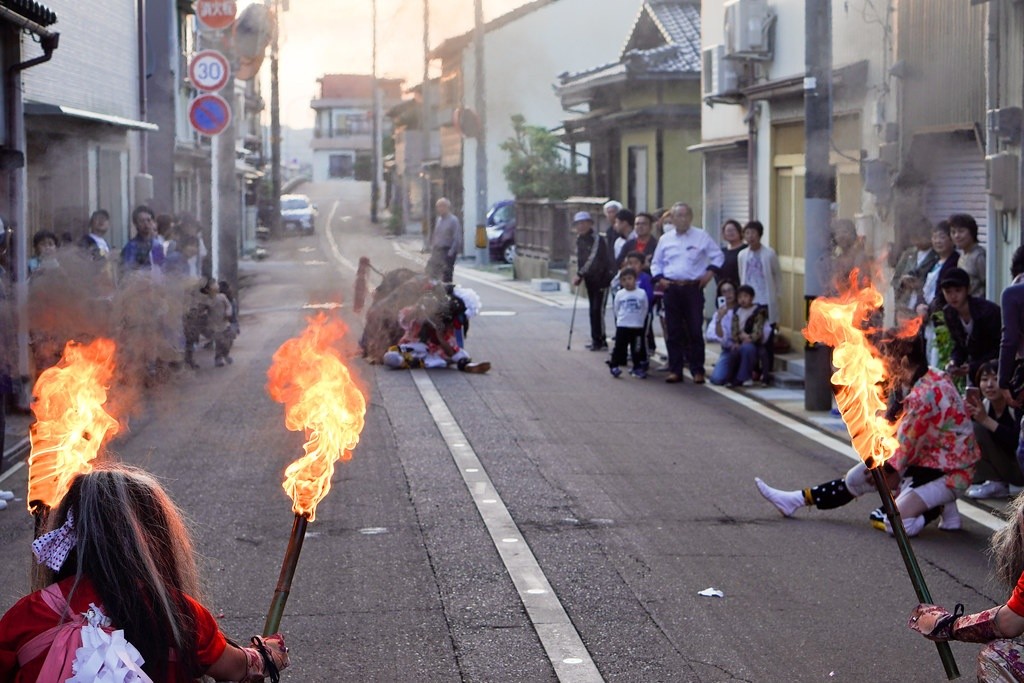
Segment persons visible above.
[907,486,1024,683]
[423,197,465,297]
[831,213,1024,500]
[569,200,783,388]
[754,326,982,538]
[0,451,292,683]
[0,205,241,511]
[358,256,492,373]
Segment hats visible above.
[571,211,594,228]
[939,267,970,288]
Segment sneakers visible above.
[463,360,490,374]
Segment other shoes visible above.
[666,373,682,382]
[0,489,14,510]
[1009,483,1024,496]
[965,479,1008,498]
[214,354,232,367]
[647,351,655,357]
[694,372,705,383]
[610,367,647,378]
[585,344,608,351]
[726,369,768,388]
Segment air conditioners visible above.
[701,46,737,98]
[723,0,769,57]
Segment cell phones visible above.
[966,386,980,407]
[718,296,726,308]
